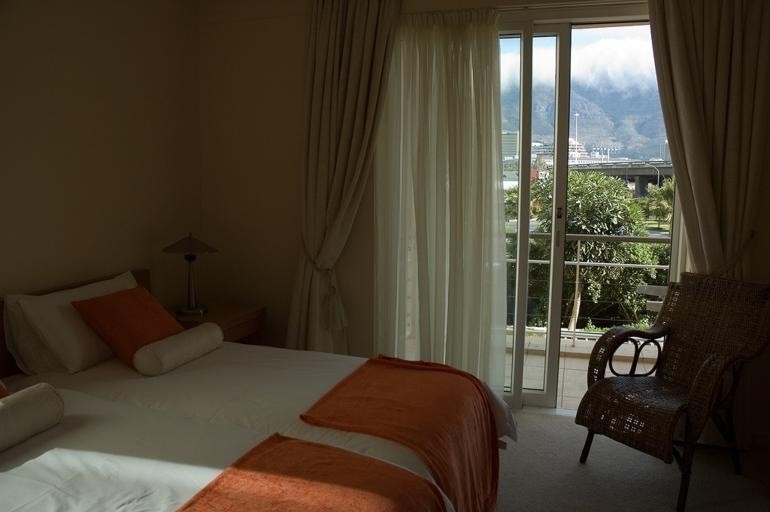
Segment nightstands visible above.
[174,303,267,345]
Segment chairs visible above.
[573,272,770,512]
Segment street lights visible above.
[646,164,659,188]
[574,113,579,160]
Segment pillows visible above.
[1,269,224,377]
[0,382,66,457]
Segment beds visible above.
[0,333,502,511]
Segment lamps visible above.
[160,232,219,321]
